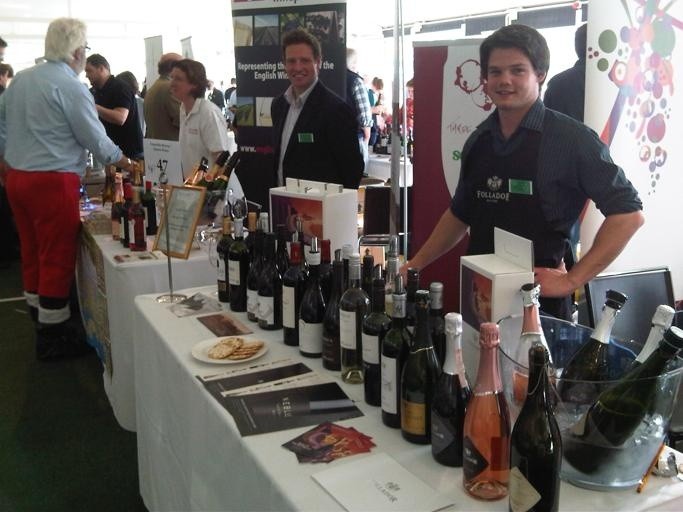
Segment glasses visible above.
[85,46,90,51]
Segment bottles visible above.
[194,151,229,192]
[376,121,416,158]
[374,93,384,116]
[185,156,208,187]
[199,151,241,223]
[215,210,681,512]
[109,171,157,252]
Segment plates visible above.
[192,334,269,364]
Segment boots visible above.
[23,291,89,359]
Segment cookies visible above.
[207,337,264,363]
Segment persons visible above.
[1,18,143,365]
[541,22,588,262]
[267,25,365,194]
[84,53,244,216]
[343,46,413,177]
[0,40,13,88]
[396,22,647,321]
